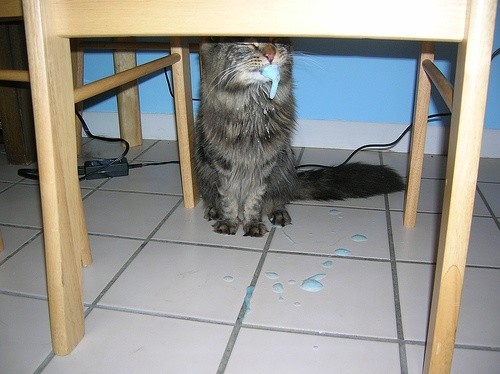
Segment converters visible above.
[85,157,131,179]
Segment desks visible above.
[20,0,498,374]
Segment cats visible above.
[190,42,407,238]
